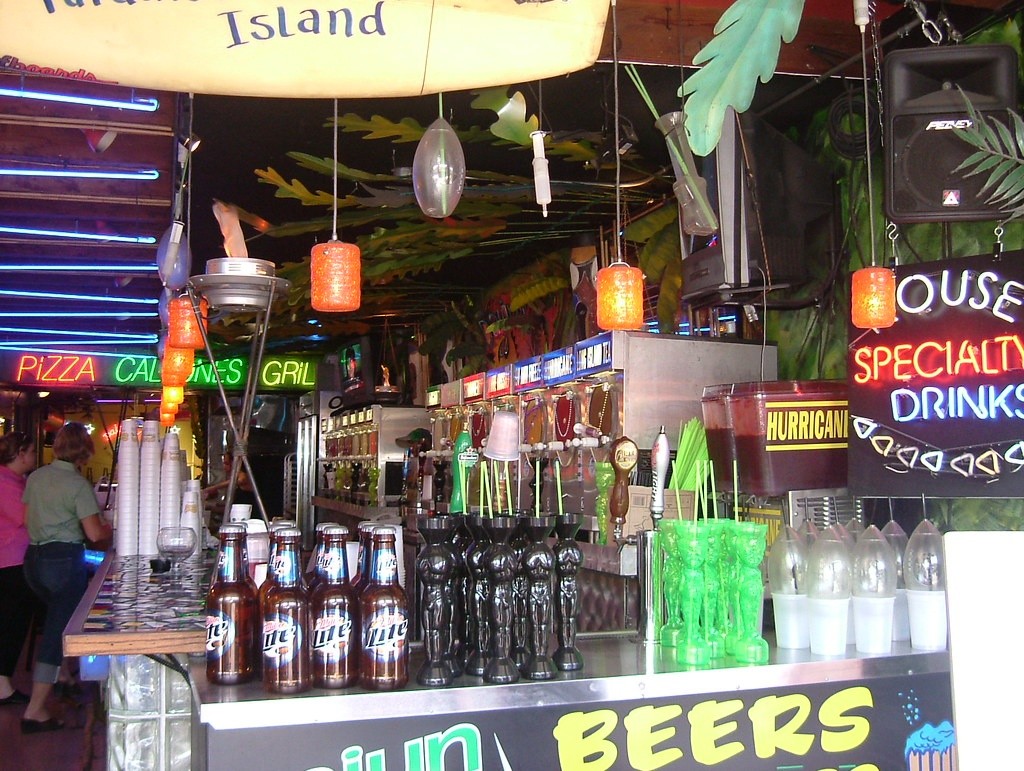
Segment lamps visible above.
[163,337,195,374]
[179,130,202,152]
[161,396,178,414]
[162,359,187,387]
[850,0,897,330]
[166,92,210,350]
[160,421,174,426]
[157,226,194,290]
[309,99,361,314]
[597,0,644,333]
[163,385,185,405]
[160,410,176,422]
[411,92,466,219]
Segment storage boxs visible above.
[726,379,850,497]
[699,383,739,492]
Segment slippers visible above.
[21,717,64,733]
[54,682,81,694]
[0,689,30,704]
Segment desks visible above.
[61,550,208,685]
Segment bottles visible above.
[206,522,258,685]
[573,386,612,437]
[326,429,378,457]
[351,521,408,691]
[526,394,542,443]
[556,391,576,441]
[434,396,521,451]
[531,436,616,515]
[304,523,356,688]
[258,517,313,693]
[449,422,473,513]
[423,444,534,511]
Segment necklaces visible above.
[557,448,576,466]
[590,448,609,463]
[472,414,484,436]
[523,400,544,443]
[597,390,610,429]
[451,420,460,442]
[555,398,573,437]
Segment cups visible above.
[483,411,519,461]
[116,420,161,556]
[851,595,897,654]
[908,591,947,650]
[161,433,203,556]
[809,597,856,656]
[327,472,336,489]
[771,593,809,648]
[230,504,252,524]
[892,589,911,642]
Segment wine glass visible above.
[157,527,198,578]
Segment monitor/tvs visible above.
[884,44,1023,224]
[678,105,842,302]
[336,335,376,405]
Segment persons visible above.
[346,347,356,381]
[204,454,254,516]
[0,432,36,707]
[24,423,110,732]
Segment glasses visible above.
[21,432,27,443]
